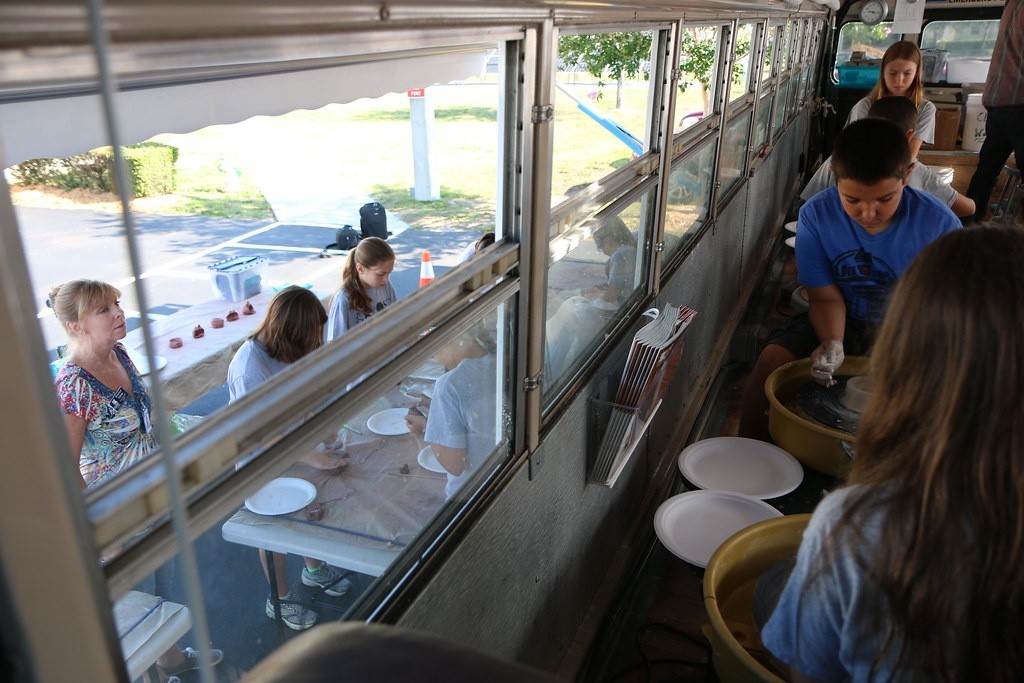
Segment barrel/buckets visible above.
[961,93,987,152]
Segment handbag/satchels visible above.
[336,225,357,249]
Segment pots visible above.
[702,514,817,683]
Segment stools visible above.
[992,162,1020,224]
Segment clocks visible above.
[859,0,889,27]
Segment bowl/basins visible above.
[765,355,870,476]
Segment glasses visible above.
[596,241,604,252]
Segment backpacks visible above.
[358,202,392,240]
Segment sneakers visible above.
[301,560,353,596]
[160,647,224,683]
[265,591,320,631]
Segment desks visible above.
[121,291,334,430]
[221,382,448,643]
[112,591,193,683]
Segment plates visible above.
[678,436,803,498]
[417,443,449,473]
[367,408,412,435]
[136,356,167,374]
[654,491,785,568]
[245,478,317,515]
[784,221,798,233]
[785,236,796,247]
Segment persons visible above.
[719,38,977,436]
[592,216,639,308]
[456,232,494,262]
[403,316,501,502]
[965,0,1024,228]
[329,237,417,391]
[761,221,1024,683]
[225,287,353,631]
[45,280,224,683]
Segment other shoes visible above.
[990,198,1016,216]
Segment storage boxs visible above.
[836,50,883,90]
[919,110,962,151]
[206,253,270,304]
